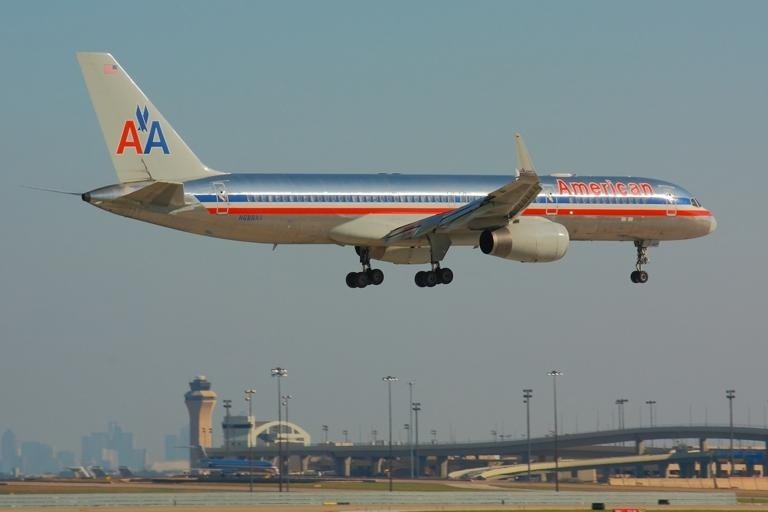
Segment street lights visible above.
[646,399,656,425]
[727,390,736,451]
[547,368,563,491]
[413,402,421,479]
[202,367,293,493]
[383,376,401,492]
[322,424,378,446]
[430,429,437,445]
[616,398,628,430]
[404,423,410,445]
[523,388,533,482]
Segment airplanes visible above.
[175,444,281,470]
[75,51,719,288]
